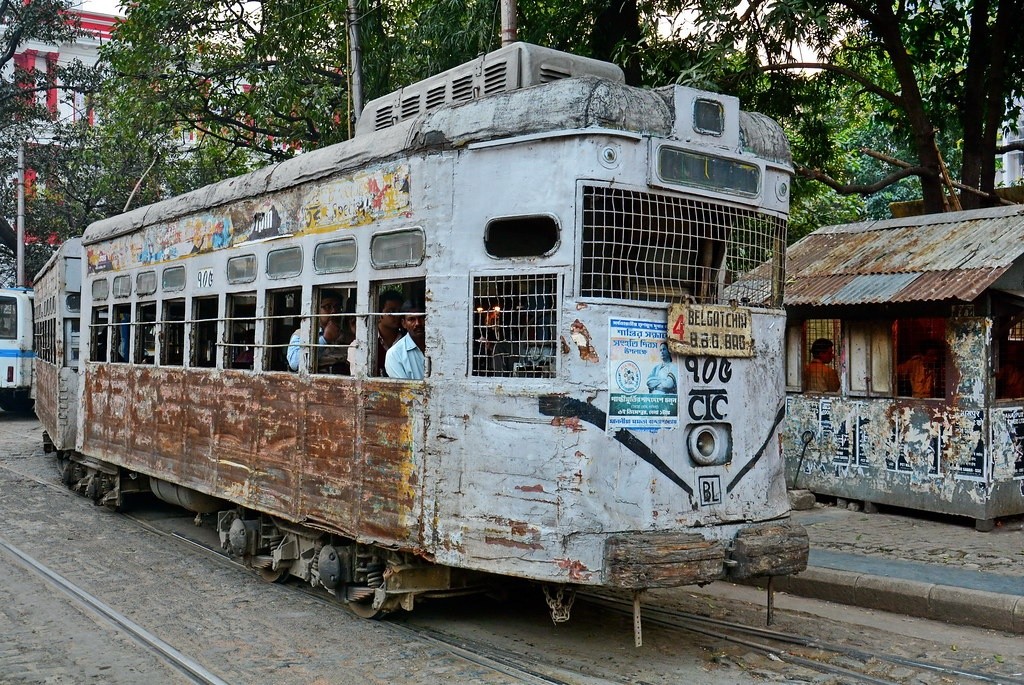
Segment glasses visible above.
[320,303,344,311]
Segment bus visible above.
[32,40,812,650]
[0,285,35,414]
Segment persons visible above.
[96,290,425,380]
[646,342,678,396]
[806,338,946,399]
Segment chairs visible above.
[96,325,387,376]
